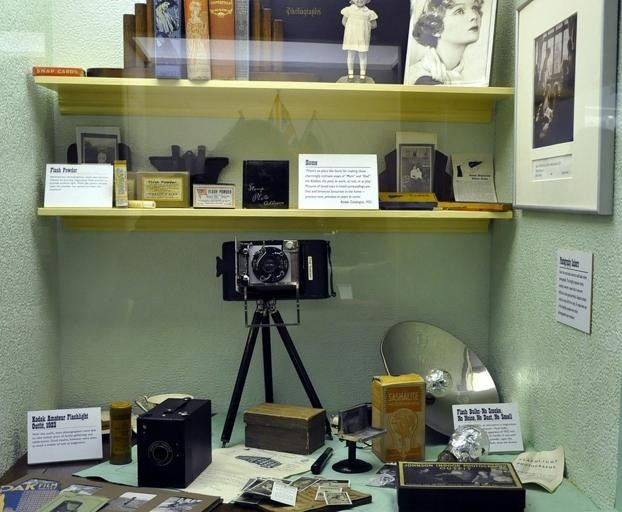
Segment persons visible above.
[534,35,576,148]
[408,0,487,84]
[340,0,378,81]
[328,493,348,505]
[95,148,109,163]
[254,482,272,495]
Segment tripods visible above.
[220,301,333,448]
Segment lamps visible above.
[380,320,499,438]
[437,425,491,462]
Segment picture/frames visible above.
[512,0,619,217]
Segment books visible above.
[208,1,236,81]
[234,1,250,82]
[152,1,186,81]
[182,0,212,82]
[261,1,408,84]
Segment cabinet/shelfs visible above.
[33,74,518,236]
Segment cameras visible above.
[215,239,336,301]
